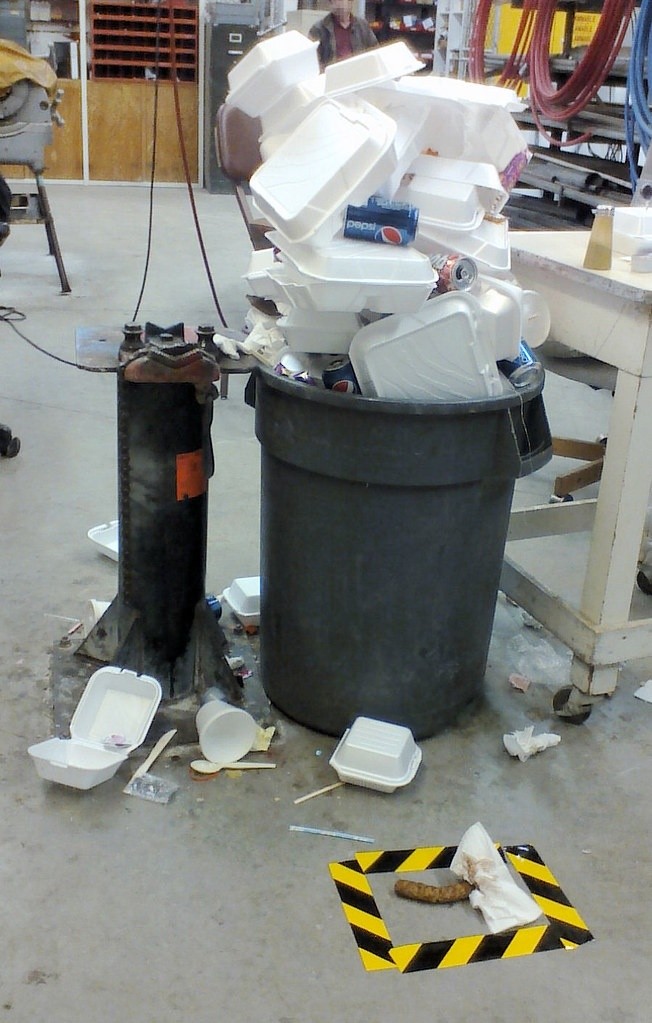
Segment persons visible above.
[309,0,381,74]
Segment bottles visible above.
[584,204,615,270]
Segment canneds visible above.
[496,336,542,387]
[428,252,478,298]
[205,593,223,621]
[341,196,420,245]
[273,362,317,385]
[322,357,361,394]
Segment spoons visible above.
[190,760,276,774]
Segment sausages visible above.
[394,879,473,903]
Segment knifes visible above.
[123,729,177,793]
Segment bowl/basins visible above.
[197,699,257,765]
